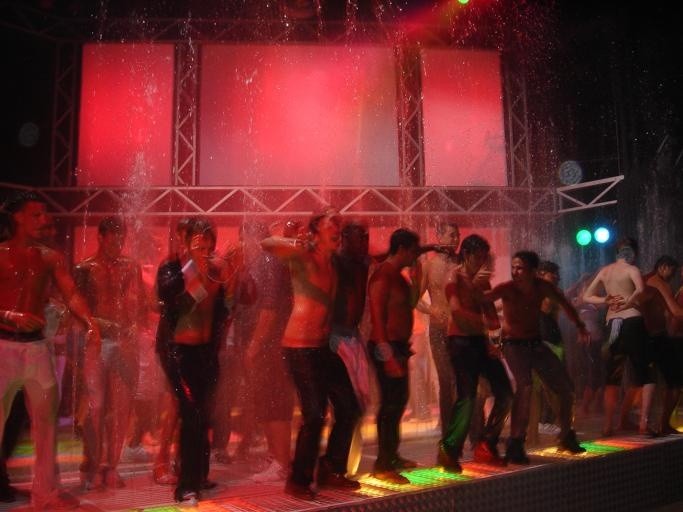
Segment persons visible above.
[617,255,683,439]
[0,203,68,504]
[436,233,514,475]
[259,204,361,503]
[149,208,296,502]
[481,250,592,465]
[415,221,485,452]
[565,269,603,421]
[582,238,657,439]
[69,214,148,490]
[365,226,422,486]
[0,190,80,511]
[333,224,454,480]
[658,284,683,436]
[526,260,562,448]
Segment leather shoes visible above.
[285,475,316,500]
[318,469,361,489]
[393,454,421,473]
[367,467,411,484]
[435,452,461,474]
[474,450,509,468]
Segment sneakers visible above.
[102,469,126,491]
[123,446,153,462]
[252,463,284,483]
[151,460,176,485]
[254,454,277,472]
[176,489,199,506]
[198,475,224,500]
[537,422,561,434]
[30,487,80,510]
[80,472,102,491]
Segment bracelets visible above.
[3,308,11,323]
